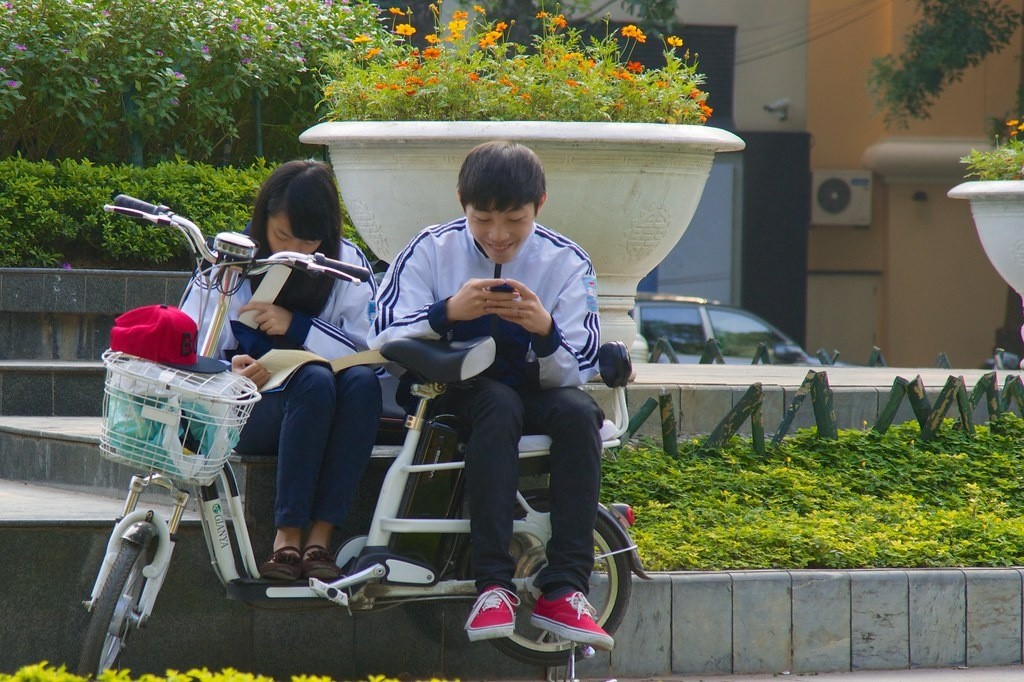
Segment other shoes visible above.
[301,543,341,582]
[258,544,302,582]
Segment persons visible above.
[177,161,383,582]
[367,141,614,652]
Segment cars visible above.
[630,291,818,364]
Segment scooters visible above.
[75,194,651,682]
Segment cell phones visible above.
[490,283,514,293]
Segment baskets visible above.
[99,347,263,486]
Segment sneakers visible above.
[464,585,521,643]
[530,592,614,652]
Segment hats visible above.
[109,305,226,375]
